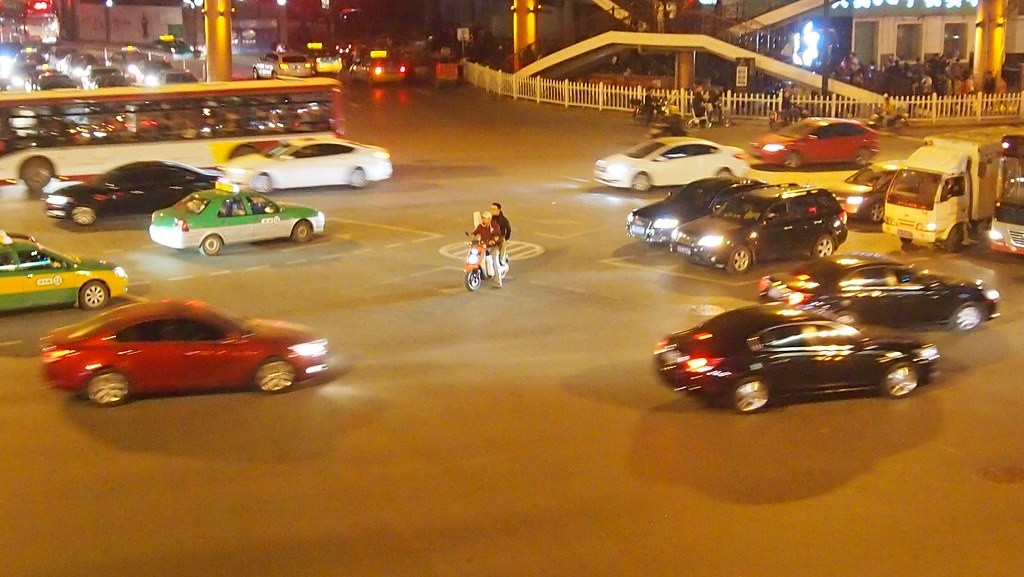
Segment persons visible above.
[642,88,657,120]
[781,91,796,124]
[250,196,264,213]
[491,203,512,266]
[694,86,720,121]
[469,211,502,287]
[812,52,996,111]
[878,93,897,127]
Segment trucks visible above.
[881,125,1024,253]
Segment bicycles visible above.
[686,105,731,128]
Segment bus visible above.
[0,5,60,50]
[984,131,1024,260]
[0,77,346,190]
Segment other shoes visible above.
[500,259,506,265]
[493,278,502,287]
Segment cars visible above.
[750,117,882,170]
[40,300,328,407]
[296,49,343,75]
[349,56,408,84]
[625,177,765,247]
[653,304,941,413]
[133,39,194,60]
[149,189,325,257]
[0,232,129,311]
[225,136,393,195]
[833,159,906,223]
[595,136,750,193]
[44,160,227,227]
[0,46,122,92]
[757,254,1002,333]
[105,50,200,85]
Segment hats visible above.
[482,211,492,218]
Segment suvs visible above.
[253,51,314,81]
[669,182,848,276]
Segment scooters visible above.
[770,106,810,129]
[465,231,509,291]
[630,97,673,126]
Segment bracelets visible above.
[492,239,495,241]
